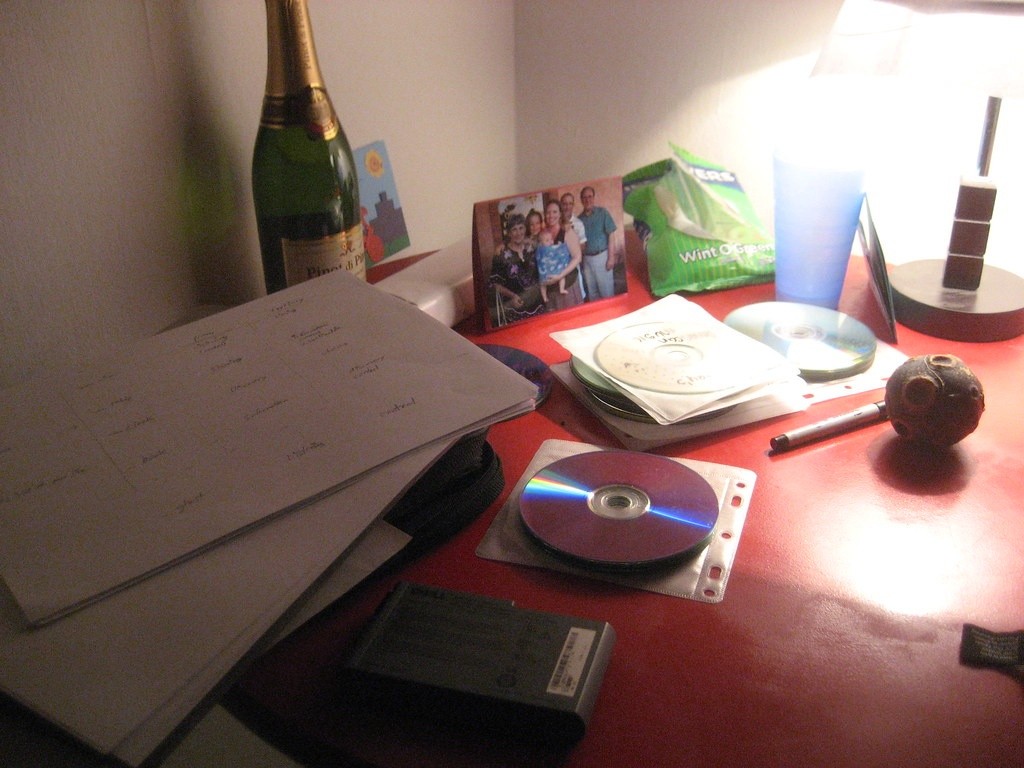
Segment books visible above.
[0,270,539,768]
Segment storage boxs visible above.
[372,234,476,327]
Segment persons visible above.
[490,186,617,326]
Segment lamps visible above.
[871,1,1024,342]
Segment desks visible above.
[220,230,1023,768]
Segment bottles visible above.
[251,0,367,295]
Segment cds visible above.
[567,299,876,426]
[520,450,719,574]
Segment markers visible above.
[768,399,888,454]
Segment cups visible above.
[774,168,864,310]
